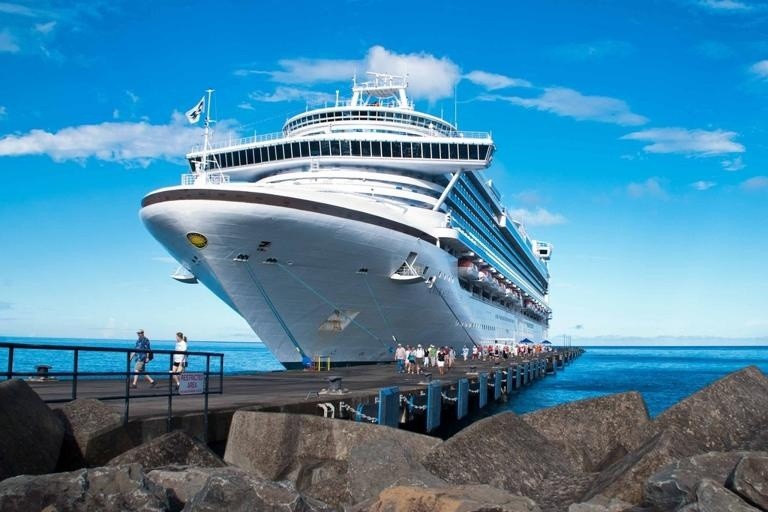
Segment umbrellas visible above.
[519,338,534,345]
[541,340,552,344]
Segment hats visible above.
[398,343,453,350]
[136,328,143,333]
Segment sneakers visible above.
[149,382,156,387]
[176,386,179,390]
[129,384,136,388]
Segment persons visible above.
[128,329,157,389]
[394,341,553,376]
[170,332,188,389]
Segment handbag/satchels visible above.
[149,353,153,359]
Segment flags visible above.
[185,88,215,124]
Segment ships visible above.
[138,68,553,363]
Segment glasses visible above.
[138,333,142,334]
